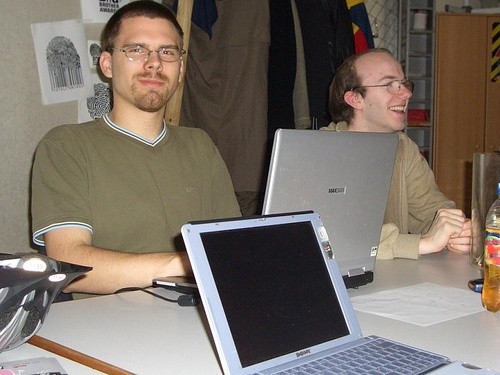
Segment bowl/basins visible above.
[0,249,94,350]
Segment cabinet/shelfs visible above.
[434,14,500,211]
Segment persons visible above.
[319,49,481,259]
[29,0,241,301]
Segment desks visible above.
[0,250,500,375]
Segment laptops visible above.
[152,128,400,294]
[180,208,449,375]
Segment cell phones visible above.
[468,278,484,292]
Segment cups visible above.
[470,152,500,269]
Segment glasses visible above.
[108,43,188,63]
[351,78,416,96]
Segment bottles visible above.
[481,182,500,312]
